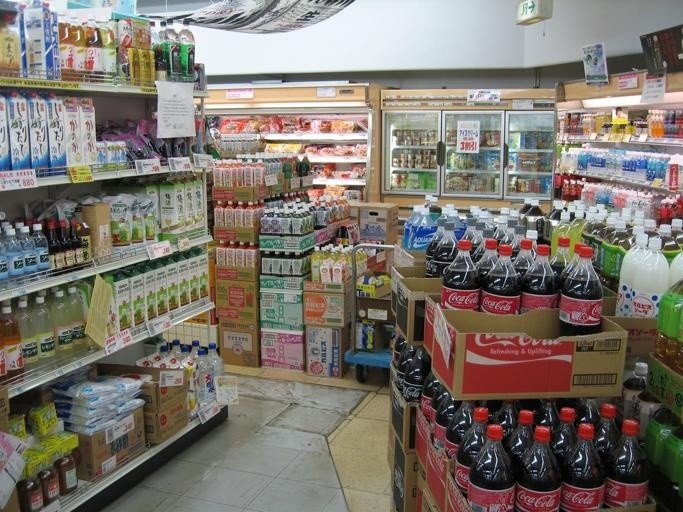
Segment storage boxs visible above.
[52,407,145,481]
[388,243,658,509]
[218,273,386,383]
[79,356,190,414]
[141,396,187,447]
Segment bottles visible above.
[396,192,682,378]
[158,337,224,408]
[55,15,118,85]
[554,107,683,227]
[0,205,91,386]
[620,357,682,510]
[383,335,648,512]
[206,151,310,186]
[214,189,354,236]
[212,239,368,284]
[335,224,349,246]
[0,12,20,77]
[149,17,195,83]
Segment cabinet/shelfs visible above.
[561,113,682,230]
[209,108,378,193]
[381,107,502,198]
[504,109,554,200]
[0,69,217,512]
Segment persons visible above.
[612,107,628,124]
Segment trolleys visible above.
[344,242,396,383]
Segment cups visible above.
[399,149,437,170]
